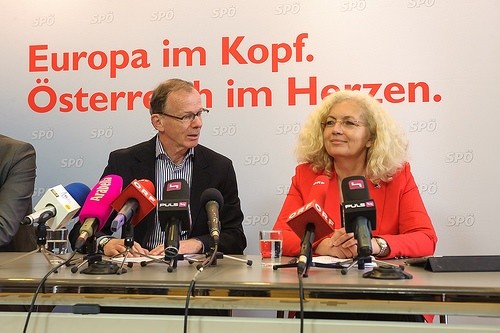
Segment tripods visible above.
[272,252,348,278]
[0,221,70,273]
[333,255,405,275]
[140,250,216,273]
[67,233,133,273]
[109,230,176,274]
[189,238,253,270]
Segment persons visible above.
[0,133,37,253]
[269,89,438,323]
[68,78,248,317]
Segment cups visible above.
[259,230,283,268]
[45,228,68,269]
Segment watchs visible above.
[376,237,388,257]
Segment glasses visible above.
[157,107,209,124]
[321,118,366,127]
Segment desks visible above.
[0,251,500,333]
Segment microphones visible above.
[19,182,91,231]
[341,175,374,257]
[200,189,225,243]
[75,174,123,250]
[286,176,335,269]
[158,179,192,254]
[110,180,158,247]
